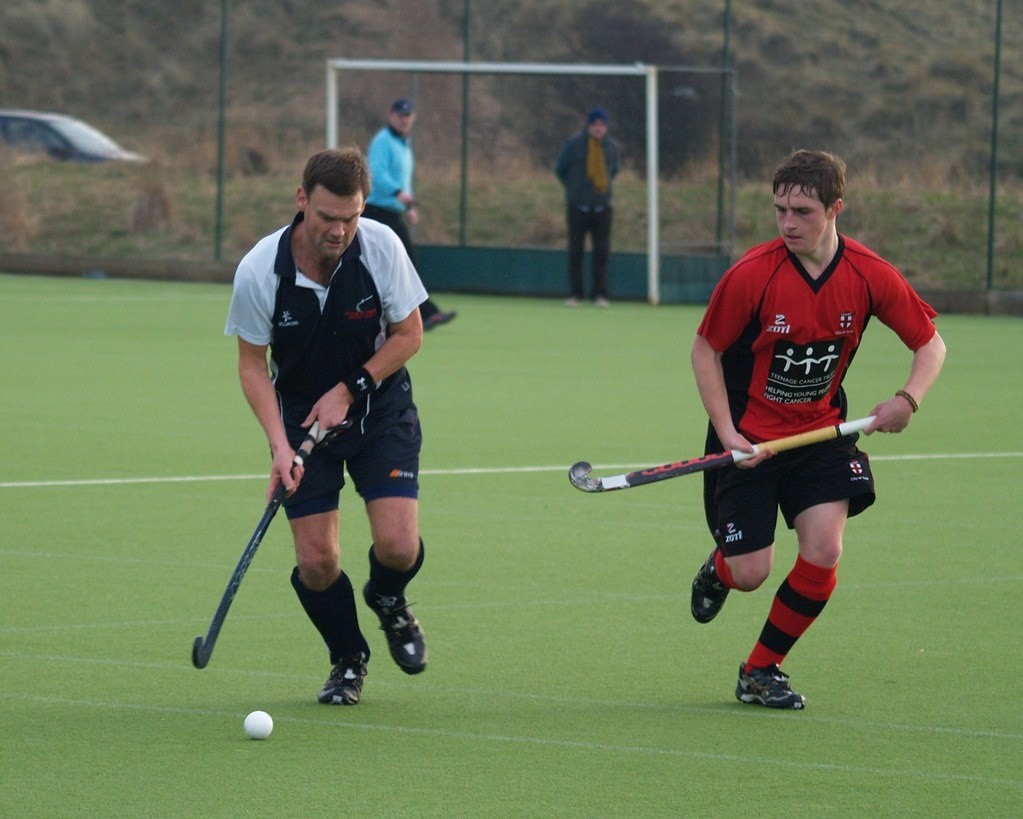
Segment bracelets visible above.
[342,366,375,402]
[896,390,919,412]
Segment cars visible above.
[0,109,142,164]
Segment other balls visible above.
[243,708,276,740]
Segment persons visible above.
[361,99,458,331]
[689,149,947,709]
[554,108,622,308]
[223,148,430,706]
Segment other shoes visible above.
[565,297,581,306]
[422,311,458,330]
[593,297,607,306]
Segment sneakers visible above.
[735,662,806,708]
[363,582,426,674]
[318,640,369,706]
[691,545,730,623]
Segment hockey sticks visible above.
[260,447,267,450]
[567,415,883,495]
[191,410,324,670]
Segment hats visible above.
[586,106,609,124]
[391,98,413,115]
